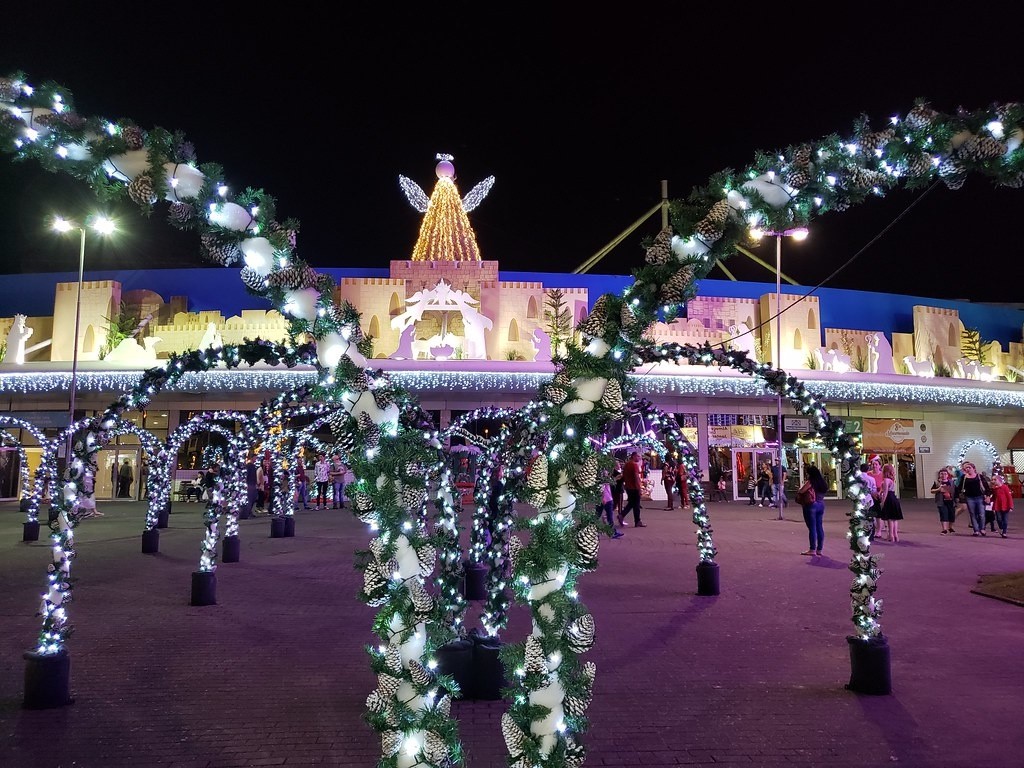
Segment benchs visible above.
[174,481,204,502]
[677,482,723,502]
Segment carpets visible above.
[970,569,1024,607]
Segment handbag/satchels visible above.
[795,486,816,504]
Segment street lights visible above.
[51,218,115,465]
[751,229,811,521]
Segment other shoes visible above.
[94,512,105,518]
[635,521,647,527]
[981,530,986,536]
[816,548,822,555]
[1002,534,1007,538]
[617,515,623,525]
[759,504,763,508]
[612,532,623,537]
[940,530,948,535]
[875,535,881,538]
[254,502,347,514]
[990,529,999,532]
[769,503,774,507]
[84,512,93,519]
[800,551,816,555]
[683,505,690,509]
[602,515,607,524]
[785,501,789,508]
[973,532,978,536]
[949,528,955,533]
[664,507,673,511]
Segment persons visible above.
[186,471,205,503]
[858,453,904,543]
[660,453,691,509]
[795,465,831,556]
[745,458,790,509]
[294,457,313,512]
[314,454,347,510]
[239,453,274,519]
[930,462,1014,538]
[205,464,220,500]
[717,476,731,503]
[595,451,648,539]
[73,470,105,518]
[110,459,133,498]
